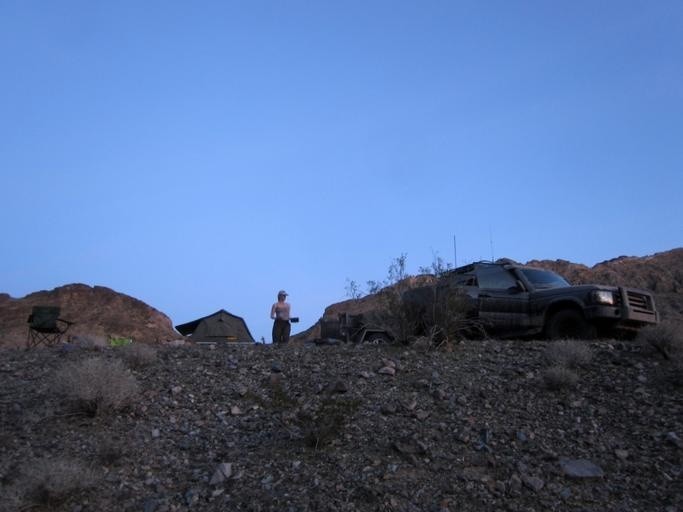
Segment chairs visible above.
[26,304,75,347]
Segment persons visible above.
[271,290,291,344]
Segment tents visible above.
[176,309,256,343]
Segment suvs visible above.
[320,310,398,345]
[401,261,659,342]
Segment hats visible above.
[279,291,289,296]
